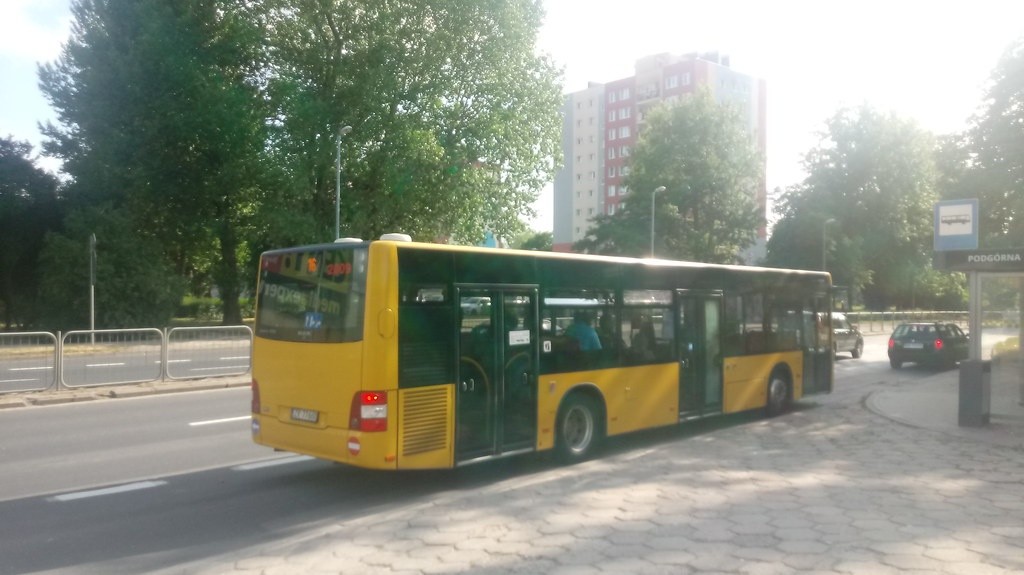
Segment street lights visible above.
[650,185,667,258]
[335,125,354,239]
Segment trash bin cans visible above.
[958,359,993,426]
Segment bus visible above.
[250,233,850,472]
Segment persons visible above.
[565,308,627,355]
[629,333,655,356]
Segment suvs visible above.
[802,309,865,359]
[889,321,971,372]
